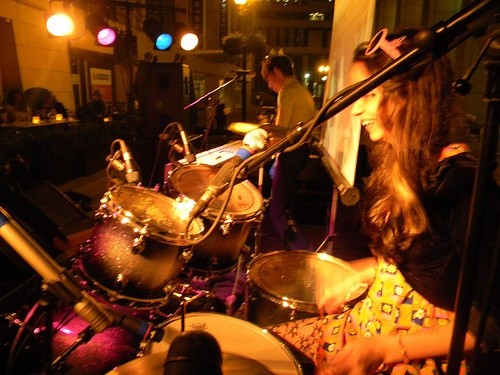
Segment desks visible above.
[0,117,82,184]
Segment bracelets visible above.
[398,334,409,364]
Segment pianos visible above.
[173,138,272,251]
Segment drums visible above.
[20,303,149,374]
[136,311,303,375]
[162,163,266,276]
[245,250,371,327]
[72,183,205,310]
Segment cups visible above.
[56,114,63,120]
[32,116,40,125]
[104,118,108,122]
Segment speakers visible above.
[4,181,93,242]
[134,62,185,140]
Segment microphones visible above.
[177,124,196,163]
[286,211,297,241]
[100,303,165,343]
[233,69,256,77]
[0,207,113,332]
[192,157,243,220]
[313,143,360,205]
[119,140,139,183]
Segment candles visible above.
[33,117,39,123]
[55,114,63,120]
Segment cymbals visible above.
[227,122,314,142]
[107,349,275,375]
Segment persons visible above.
[5,89,31,120]
[89,89,106,118]
[262,55,316,242]
[263,27,500,375]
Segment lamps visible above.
[85,14,118,47]
[44,0,73,38]
[142,18,172,51]
[174,24,198,51]
